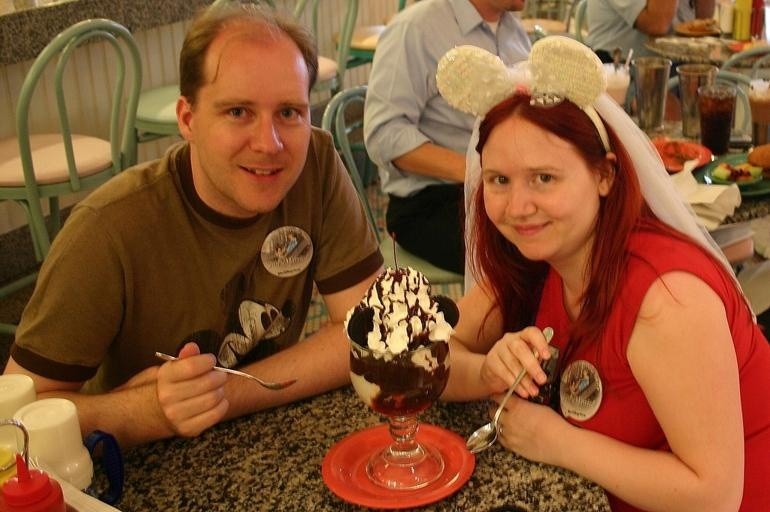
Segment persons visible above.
[1,0,402,453]
[572,0,723,67]
[362,0,537,275]
[437,90,770,509]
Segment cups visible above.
[600,59,630,106]
[678,63,718,140]
[1,373,95,493]
[632,55,672,132]
[696,83,736,156]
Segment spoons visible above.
[463,324,556,455]
[152,347,297,393]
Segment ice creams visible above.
[343,265,459,418]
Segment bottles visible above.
[718,1,765,40]
[3,458,64,512]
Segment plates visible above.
[322,424,476,509]
[674,22,720,36]
[692,155,769,198]
[654,137,713,171]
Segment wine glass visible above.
[348,337,450,489]
